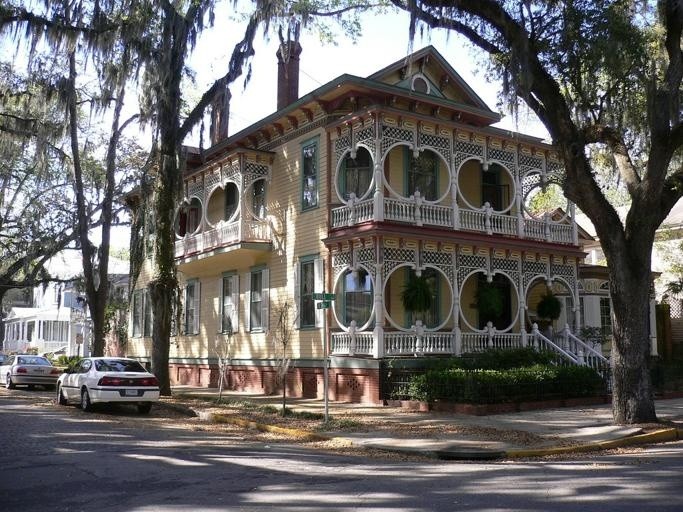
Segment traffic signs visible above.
[317,302,330,308]
[312,292,335,300]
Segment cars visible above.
[0,354,58,389]
[55,357,159,411]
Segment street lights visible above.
[75,297,87,356]
